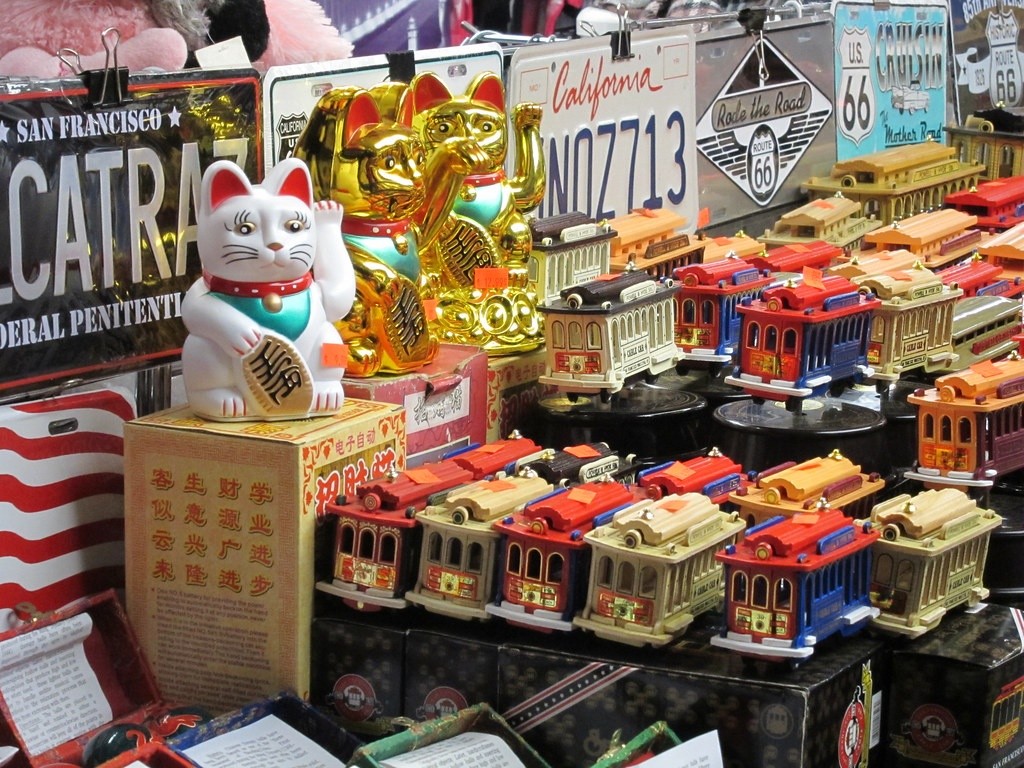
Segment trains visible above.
[311,115,1023,664]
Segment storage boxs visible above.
[0,103,1024,767]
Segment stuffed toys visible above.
[0,0,357,93]
